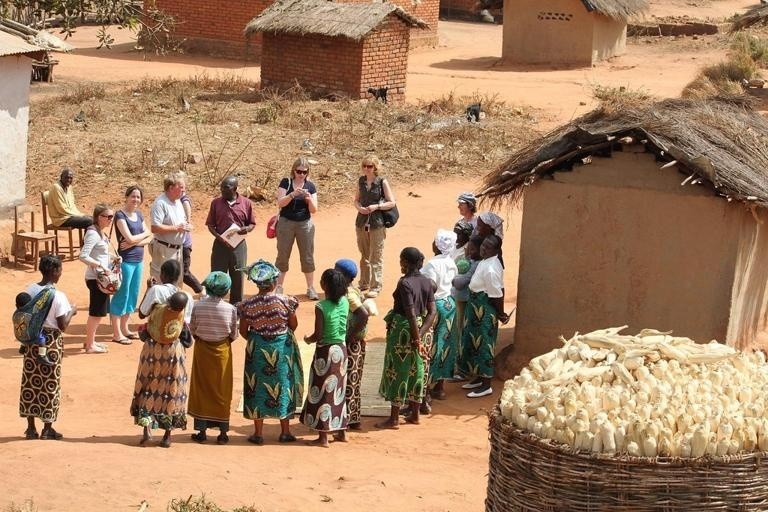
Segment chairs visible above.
[41,189,83,262]
[14,205,64,271]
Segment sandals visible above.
[466,384,494,399]
[460,376,484,389]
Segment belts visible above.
[154,237,183,250]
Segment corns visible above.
[499,324,768,457]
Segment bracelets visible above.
[290,193,295,199]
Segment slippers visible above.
[127,331,142,342]
[111,334,132,344]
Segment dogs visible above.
[465,102,481,123]
[367,87,389,104]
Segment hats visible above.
[457,191,477,206]
[433,228,458,256]
[335,257,359,278]
[201,270,233,297]
[480,210,504,231]
[243,258,280,291]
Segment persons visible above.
[182,194,209,301]
[20,254,77,440]
[237,259,303,444]
[399,193,510,414]
[16,292,55,366]
[205,176,256,306]
[150,174,192,287]
[48,169,93,246]
[188,271,239,444]
[138,292,191,348]
[130,259,195,447]
[354,154,396,297]
[78,204,123,354]
[299,260,368,448]
[373,246,437,429]
[273,156,318,300]
[110,186,151,345]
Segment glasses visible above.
[294,168,308,175]
[362,164,374,169]
[99,214,113,221]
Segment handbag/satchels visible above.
[95,262,123,296]
[265,215,279,239]
[379,196,400,229]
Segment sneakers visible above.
[353,283,381,298]
[83,341,108,354]
[401,388,449,416]
[36,354,56,367]
[139,430,153,447]
[275,287,320,301]
[24,427,39,440]
[191,431,207,443]
[248,434,266,446]
[160,434,173,448]
[279,432,297,442]
[39,427,63,440]
[216,433,229,444]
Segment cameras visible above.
[364,224,371,233]
[301,188,308,194]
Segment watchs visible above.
[378,203,381,210]
